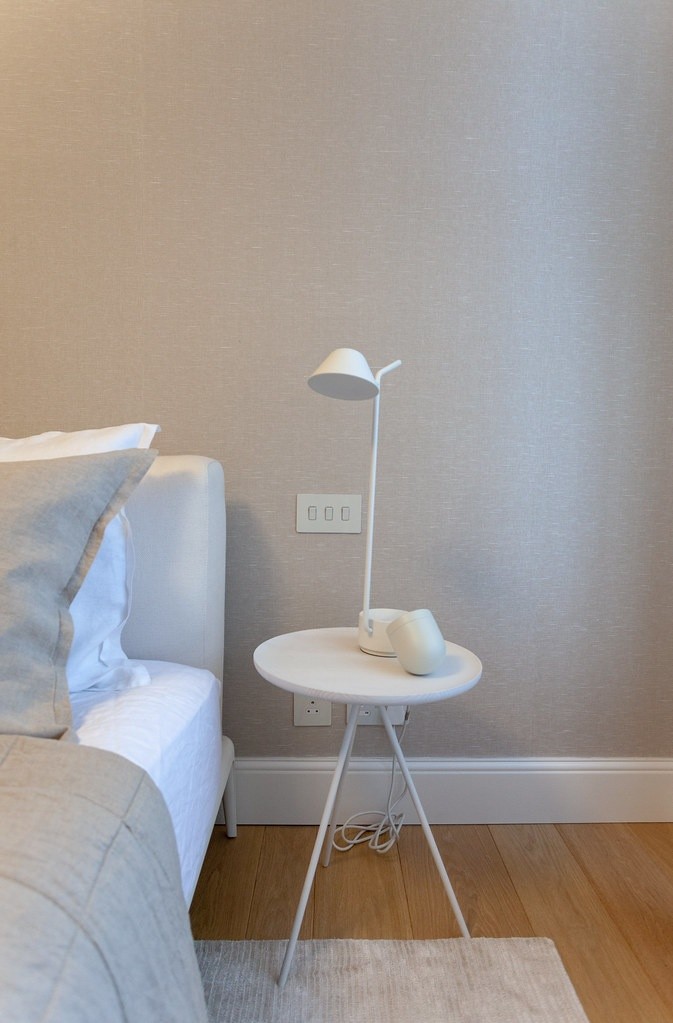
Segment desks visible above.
[254,629,482,985]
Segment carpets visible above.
[191,936,590,1022]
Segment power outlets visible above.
[345,703,407,727]
[293,692,332,726]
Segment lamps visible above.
[307,349,413,657]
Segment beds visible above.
[1,452,237,1023]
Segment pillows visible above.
[0,447,158,738]
[1,422,162,692]
